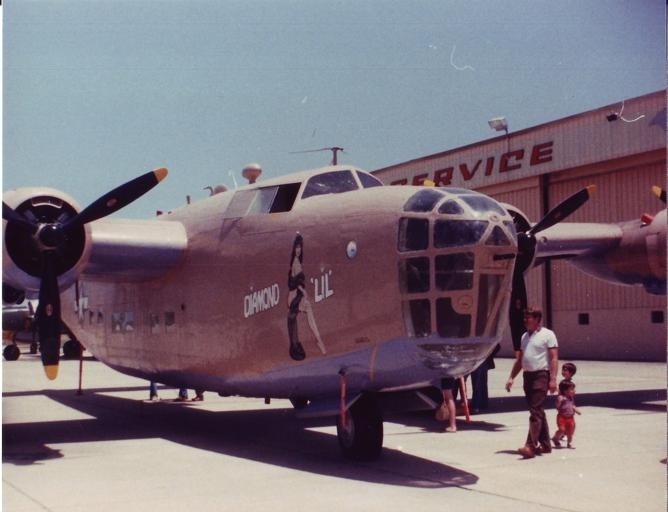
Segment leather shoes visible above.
[174,395,203,401]
[518,440,552,458]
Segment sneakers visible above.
[552,437,576,450]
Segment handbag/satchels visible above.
[435,389,450,421]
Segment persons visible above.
[441,375,470,431]
[552,362,582,448]
[471,342,501,415]
[505,308,559,459]
